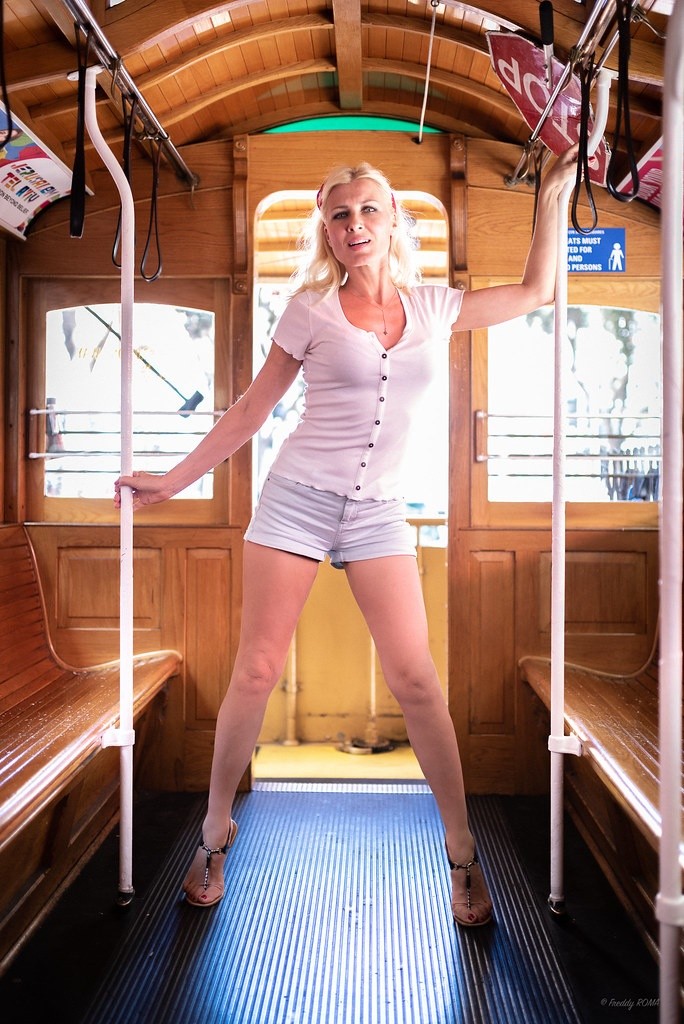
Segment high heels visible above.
[445,837,492,926]
[185,819,238,906]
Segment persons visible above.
[113,143,594,926]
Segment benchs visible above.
[0,529,189,961]
[519,614,684,891]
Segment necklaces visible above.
[344,284,396,335]
